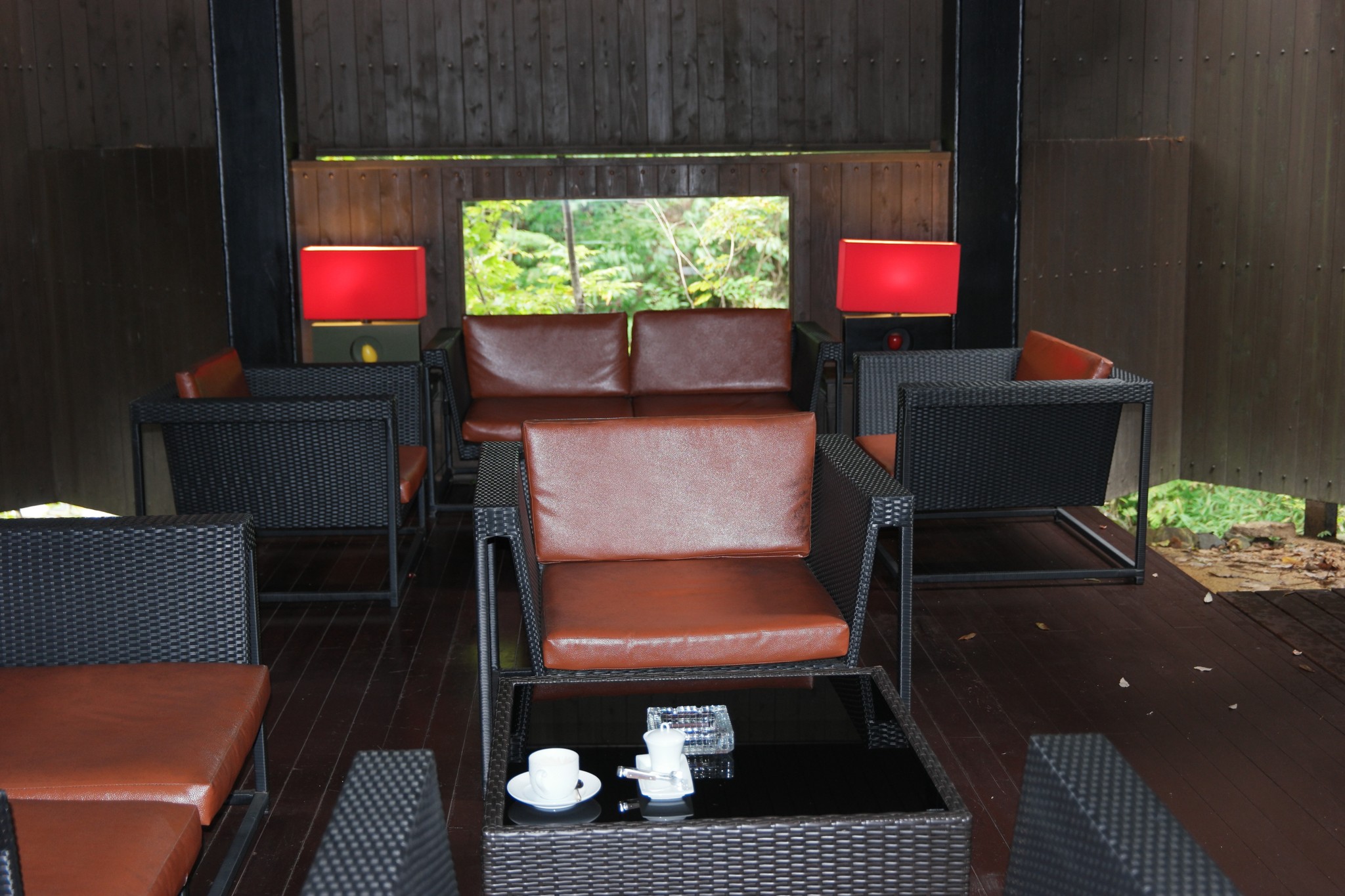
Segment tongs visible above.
[616,766,691,790]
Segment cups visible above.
[643,720,688,774]
[528,748,579,800]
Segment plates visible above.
[507,769,601,809]
[635,753,694,799]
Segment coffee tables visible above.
[488,655,976,896]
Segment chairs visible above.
[472,413,918,775]
[299,728,1241,896]
[851,319,1160,590]
[135,352,433,610]
[2,507,270,893]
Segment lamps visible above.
[832,237,960,353]
[299,241,432,364]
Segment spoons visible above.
[575,779,584,801]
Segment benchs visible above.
[424,300,844,497]
